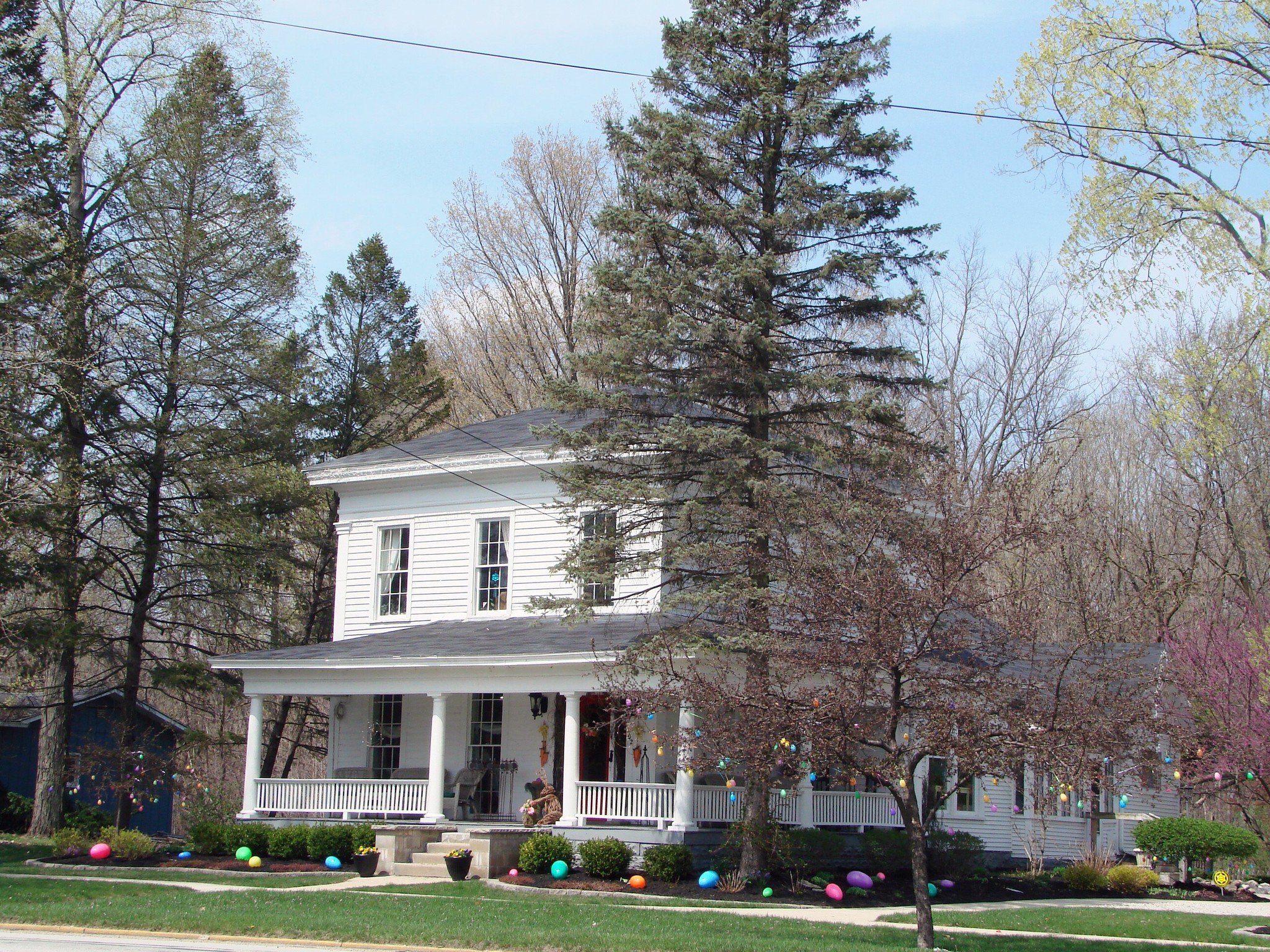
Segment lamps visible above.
[528,692,549,720]
[334,701,348,720]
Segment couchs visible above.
[665,769,712,829]
[705,774,759,787]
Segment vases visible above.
[353,852,380,877]
[443,856,473,881]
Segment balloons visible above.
[49,752,210,811]
[235,847,262,867]
[551,860,568,880]
[846,871,873,889]
[928,883,937,897]
[178,852,192,859]
[326,856,341,869]
[941,880,954,887]
[89,843,111,859]
[877,872,886,880]
[763,887,773,897]
[629,875,646,888]
[826,883,843,900]
[509,869,518,875]
[699,870,720,888]
[626,695,1253,837]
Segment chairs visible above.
[443,768,487,821]
[328,766,375,818]
[376,769,449,818]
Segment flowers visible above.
[447,848,474,858]
[354,845,379,855]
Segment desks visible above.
[930,784,971,810]
